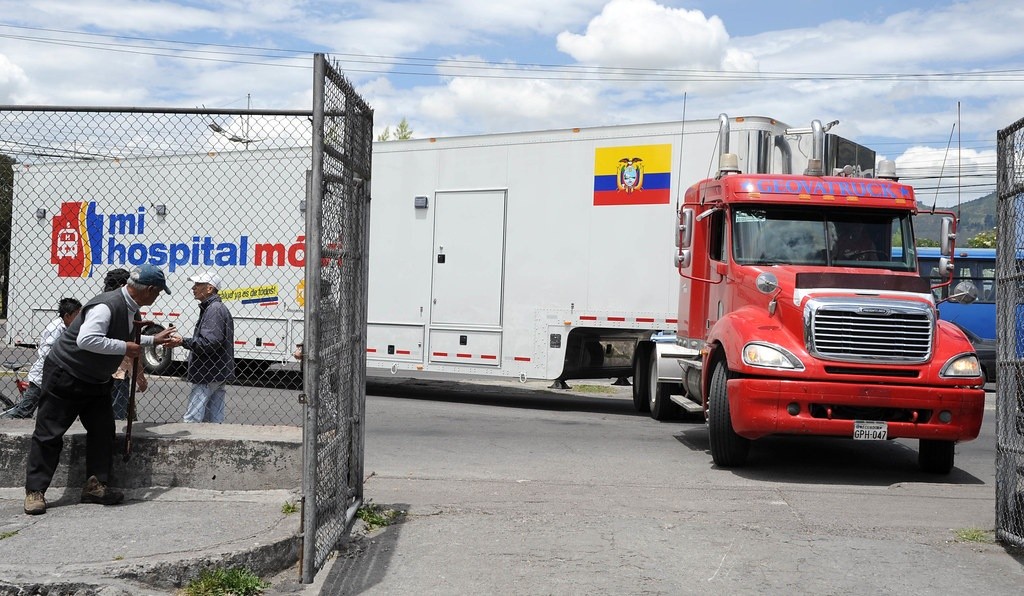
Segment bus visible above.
[891,247,1024,389]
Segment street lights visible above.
[208,93,252,150]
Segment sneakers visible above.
[24,491,47,514]
[81,476,124,505]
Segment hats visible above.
[130,264,171,295]
[187,272,222,291]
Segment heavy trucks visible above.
[5,114,986,478]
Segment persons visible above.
[162,272,234,423]
[11,264,176,513]
[832,217,876,260]
